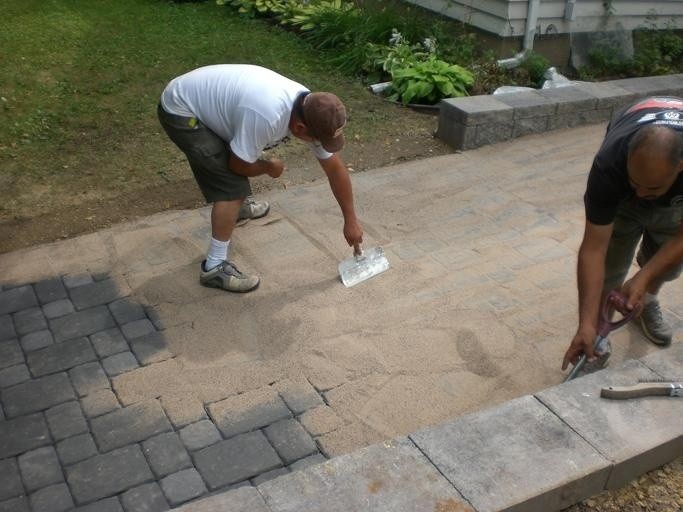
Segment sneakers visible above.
[571,339,612,378]
[199,257,260,293]
[639,300,673,345]
[235,198,270,223]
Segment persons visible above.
[561,92,683,372]
[158,62,364,294]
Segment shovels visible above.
[337,244,391,288]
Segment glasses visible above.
[308,130,321,148]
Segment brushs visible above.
[600,378,682,401]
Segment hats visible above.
[303,92,347,153]
